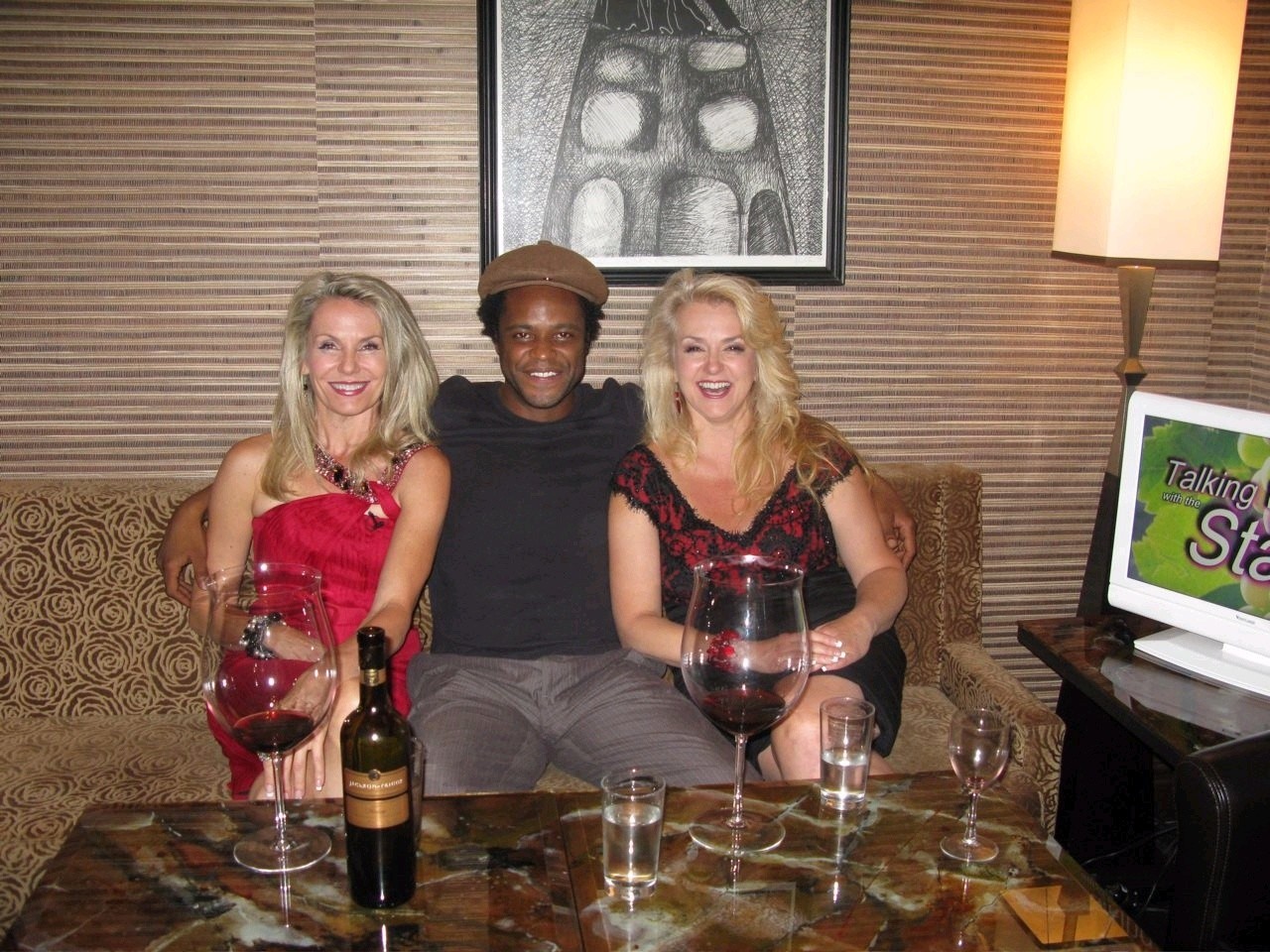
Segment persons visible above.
[189,271,454,800]
[154,240,918,798]
[609,267,908,781]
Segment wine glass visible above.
[199,561,338,874]
[680,554,810,856]
[939,707,1010,863]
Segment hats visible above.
[476,239,608,305]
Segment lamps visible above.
[1051,0,1249,614]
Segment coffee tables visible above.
[0,770,1159,952]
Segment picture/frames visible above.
[476,0,851,286]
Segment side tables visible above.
[1018,613,1270,952]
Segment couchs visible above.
[0,464,1065,952]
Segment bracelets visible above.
[238,612,287,661]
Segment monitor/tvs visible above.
[1106,391,1270,704]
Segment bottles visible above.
[340,626,416,910]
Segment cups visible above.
[600,768,666,901]
[410,736,425,842]
[819,696,876,802]
